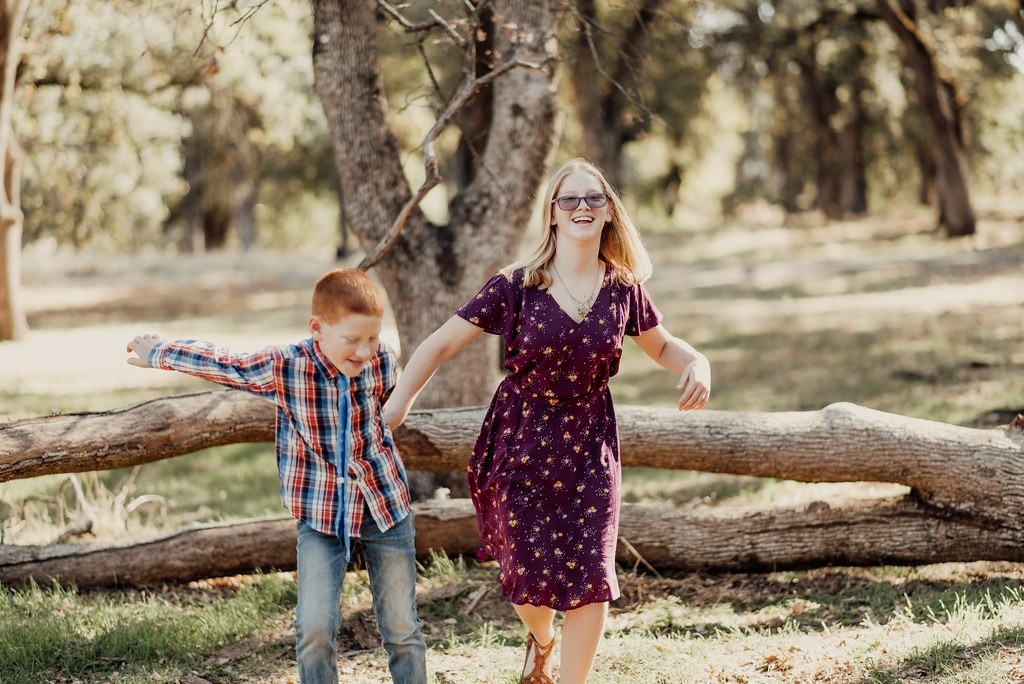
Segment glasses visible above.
[551,192,609,211]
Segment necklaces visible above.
[552,258,600,319]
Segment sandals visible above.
[518,632,555,684]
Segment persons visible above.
[381,157,711,683]
[125,268,429,684]
